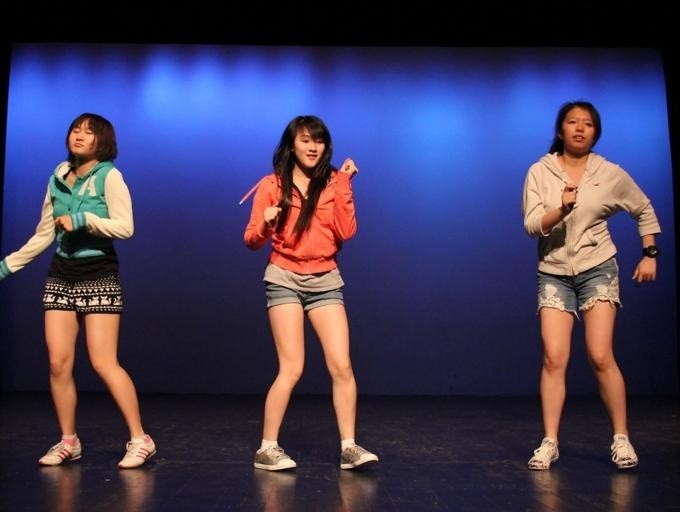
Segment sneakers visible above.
[39,440,83,466]
[611,433,639,469]
[117,434,157,469]
[341,443,379,469]
[254,444,296,471]
[528,437,559,470]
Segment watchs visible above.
[642,245,659,258]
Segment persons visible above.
[42,464,155,512]
[238,113,379,472]
[522,100,662,470]
[251,466,376,512]
[529,468,637,512]
[0,112,157,469]
